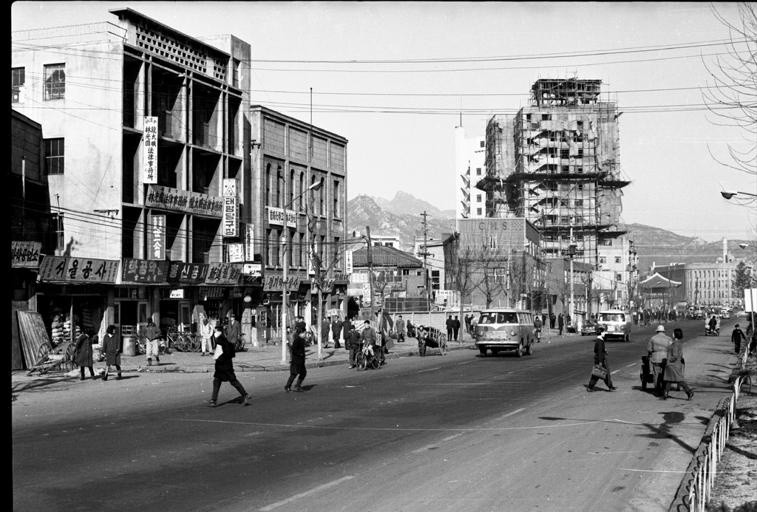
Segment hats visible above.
[654,324,666,333]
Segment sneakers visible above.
[240,393,251,406]
[662,393,670,399]
[587,387,600,392]
[79,375,122,381]
[205,396,221,408]
[608,386,618,392]
[287,385,308,393]
[685,390,694,401]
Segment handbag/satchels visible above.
[592,363,609,380]
[663,362,686,383]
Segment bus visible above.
[472,308,536,357]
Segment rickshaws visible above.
[414,325,452,356]
[163,326,201,356]
[28,337,81,380]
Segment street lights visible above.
[739,244,757,250]
[274,179,325,368]
[721,191,757,201]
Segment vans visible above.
[596,308,632,342]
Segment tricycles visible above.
[704,319,719,337]
[635,350,685,401]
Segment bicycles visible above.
[356,339,382,370]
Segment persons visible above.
[585,300,748,399]
[199,311,252,408]
[283,311,428,393]
[441,308,574,351]
[71,317,161,382]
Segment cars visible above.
[680,299,733,319]
